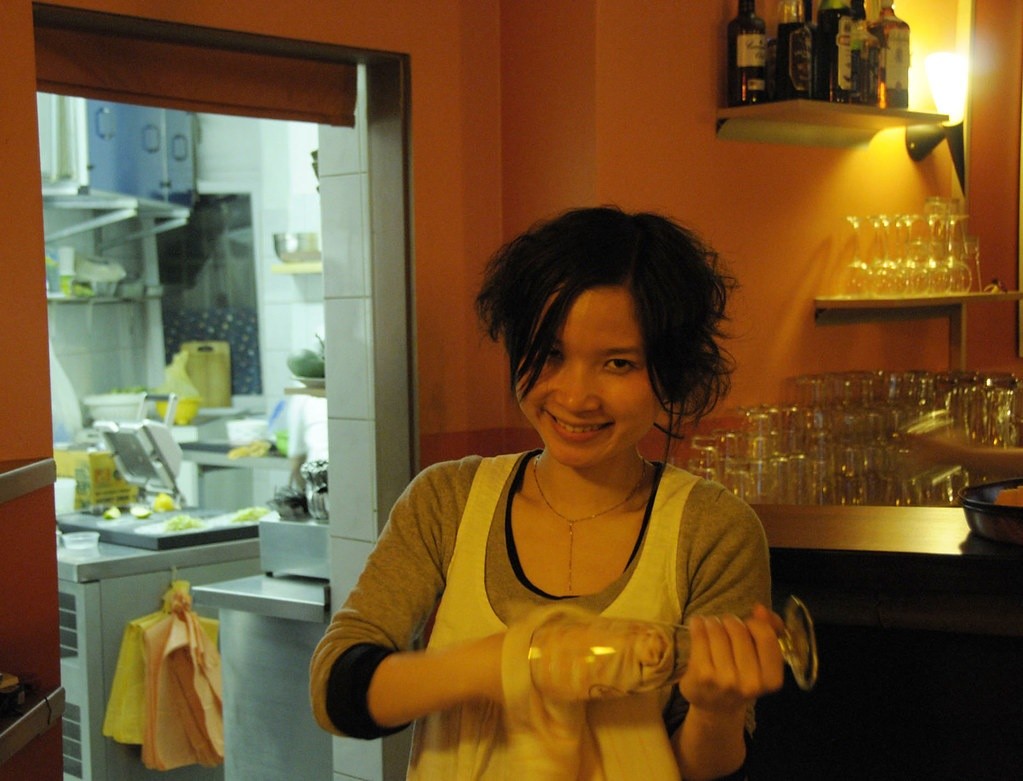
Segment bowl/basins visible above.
[62,531,100,550]
[155,400,199,424]
[228,419,268,447]
[958,477,1023,546]
[274,231,317,264]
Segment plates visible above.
[291,374,325,389]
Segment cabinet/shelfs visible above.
[717,100,1021,312]
[280,258,327,397]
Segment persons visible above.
[307,203,784,781]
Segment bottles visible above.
[728,0,911,108]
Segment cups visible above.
[669,368,1022,507]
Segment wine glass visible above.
[533,595,819,700]
[845,196,985,293]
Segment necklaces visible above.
[533,452,647,598]
